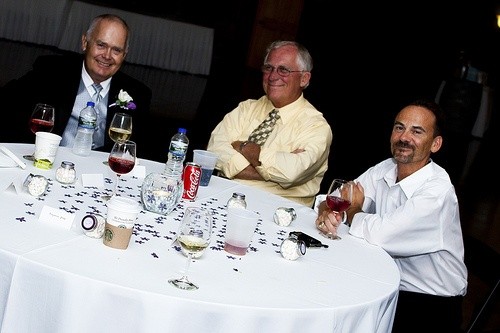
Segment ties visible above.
[247,108,279,146]
[91,83,103,103]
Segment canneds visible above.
[180,162,202,200]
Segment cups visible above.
[102,113,132,166]
[224,208,259,256]
[101,139,136,201]
[33,132,62,171]
[103,197,139,251]
[193,149,218,186]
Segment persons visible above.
[314,105,468,333]
[207,41,332,209]
[12,14,152,158]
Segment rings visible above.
[318,221,325,227]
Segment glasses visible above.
[262,64,308,77]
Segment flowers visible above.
[107,87,138,112]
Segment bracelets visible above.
[240,141,255,152]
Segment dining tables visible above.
[0,133,401,333]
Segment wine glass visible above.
[319,179,352,240]
[23,103,54,161]
[168,207,212,291]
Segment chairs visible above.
[424,231,500,333]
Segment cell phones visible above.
[289,231,321,248]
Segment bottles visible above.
[72,101,97,156]
[23,173,49,198]
[164,128,189,179]
[280,238,306,260]
[227,192,247,209]
[273,206,297,226]
[80,215,105,239]
[55,161,76,184]
